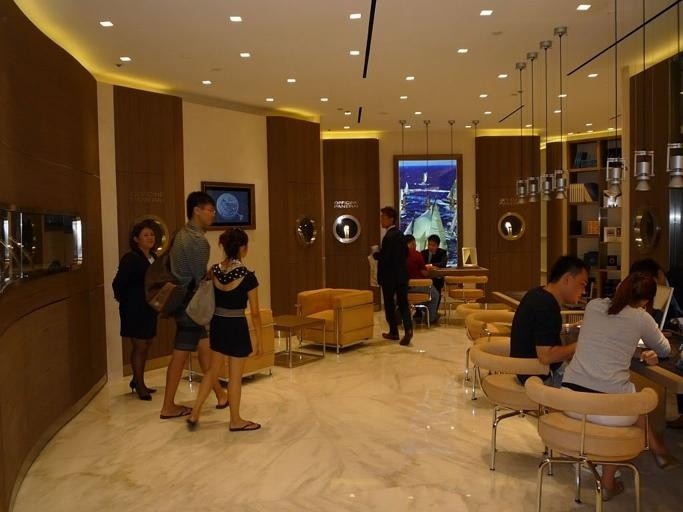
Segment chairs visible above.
[181,307,276,384]
[400,277,432,328]
[525,377,659,512]
[446,275,520,385]
[470,340,559,471]
[296,288,375,355]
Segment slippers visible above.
[216,400,260,431]
[160,406,192,419]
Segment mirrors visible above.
[0,200,88,298]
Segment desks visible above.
[629,328,683,446]
[489,287,592,335]
[274,314,326,369]
[427,266,489,278]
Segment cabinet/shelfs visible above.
[562,130,622,304]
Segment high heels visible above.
[130,380,155,400]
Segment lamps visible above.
[397,119,480,213]
[515,0,682,205]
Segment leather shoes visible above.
[382,330,412,345]
[601,482,624,501]
[656,453,680,471]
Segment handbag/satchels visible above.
[144,252,215,325]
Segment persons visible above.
[560,271,683,501]
[509,254,590,389]
[629,259,683,334]
[187,227,264,430]
[413,235,447,323]
[158,192,228,418]
[405,235,441,324]
[373,206,413,345]
[112,223,159,401]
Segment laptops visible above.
[636,282,674,351]
